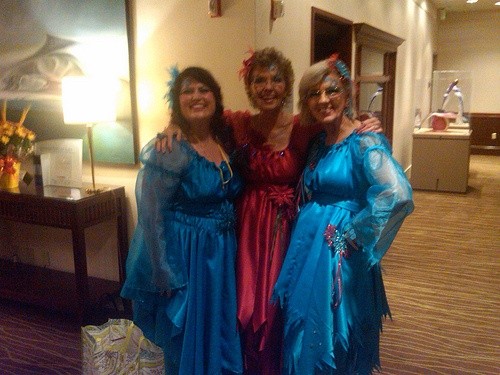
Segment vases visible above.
[0,159,21,193]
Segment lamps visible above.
[62,76,121,194]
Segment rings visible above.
[157,134,164,139]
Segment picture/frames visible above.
[0,0,139,169]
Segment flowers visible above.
[266,183,295,209]
[0,120,37,161]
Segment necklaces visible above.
[190,127,234,185]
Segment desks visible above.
[0,185,129,325]
[411,128,473,193]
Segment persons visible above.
[154,48,382,375]
[121,67,244,375]
[271,57,414,375]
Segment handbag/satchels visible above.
[80,318,165,375]
